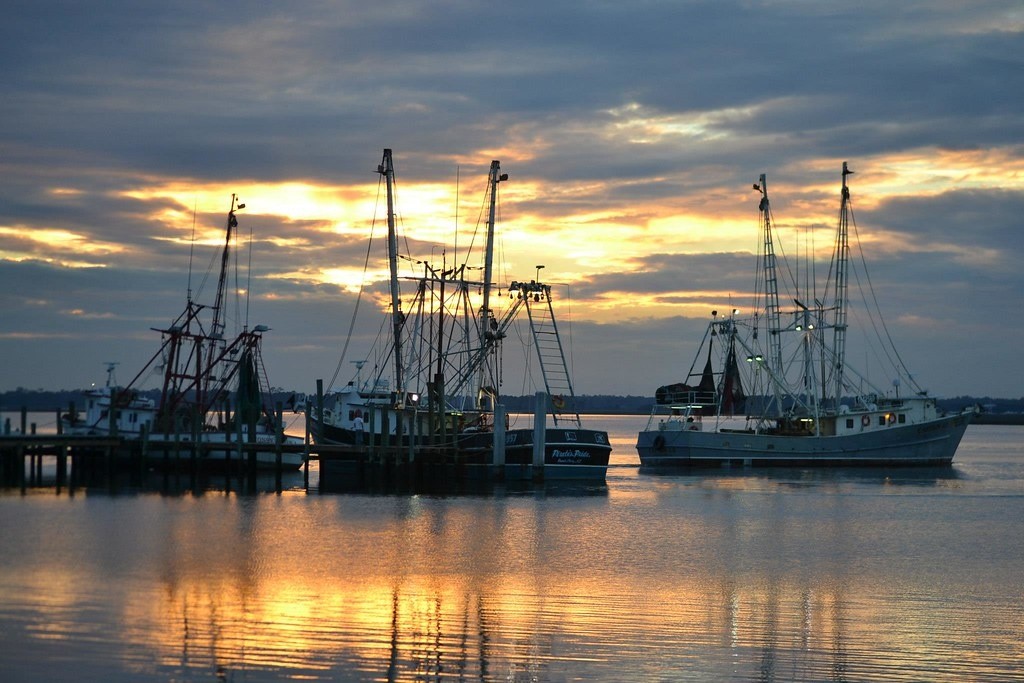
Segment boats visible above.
[632,158,974,467]
[303,148,615,485]
[58,188,306,469]
